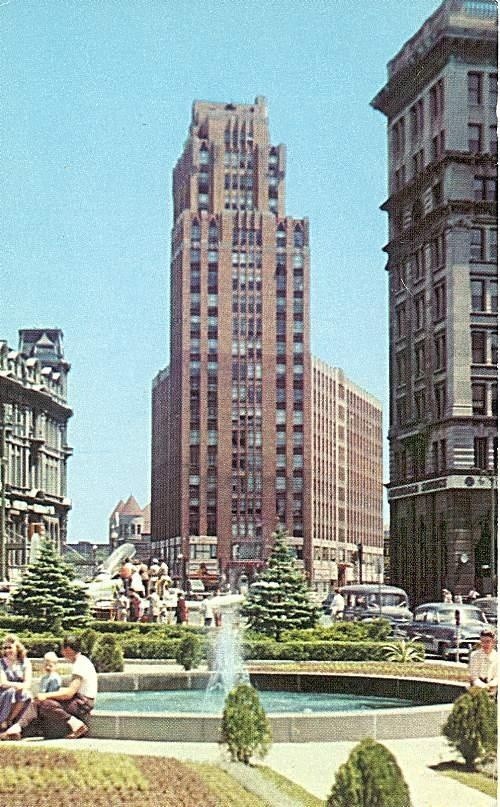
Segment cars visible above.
[396,602,494,663]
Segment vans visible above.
[339,584,414,637]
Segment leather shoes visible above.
[67,725,89,738]
[0,732,21,741]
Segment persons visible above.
[329,589,346,619]
[441,589,480,605]
[199,594,214,626]
[469,630,498,695]
[111,558,190,626]
[0,634,99,741]
